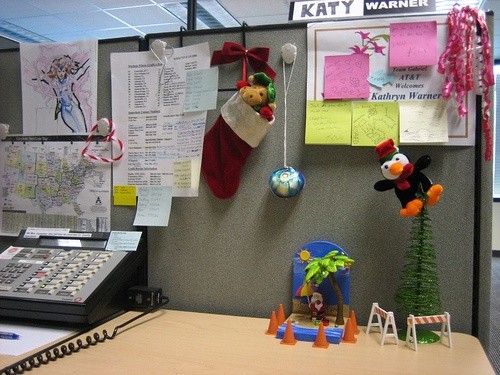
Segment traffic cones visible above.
[280,320,297,345]
[265,310,279,334]
[276,303,285,326]
[342,310,359,344]
[312,322,330,348]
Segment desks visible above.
[0,308,497,375]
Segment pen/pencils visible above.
[0,331,19,339]
